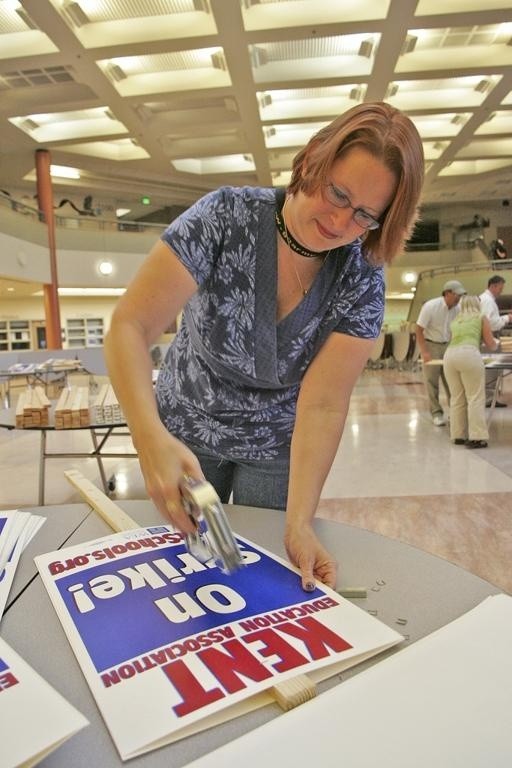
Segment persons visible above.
[416,280,466,426]
[478,274,512,407]
[442,295,500,448]
[103,101,425,591]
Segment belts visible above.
[425,339,447,344]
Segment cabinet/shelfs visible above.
[0,320,46,350]
[68,319,104,348]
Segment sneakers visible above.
[455,438,465,444]
[433,417,445,426]
[471,440,488,448]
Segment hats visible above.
[442,280,468,296]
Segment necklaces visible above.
[283,193,331,297]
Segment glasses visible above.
[324,183,380,231]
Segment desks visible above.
[1,397,139,507]
[0,500,512,768]
[2,367,83,407]
[431,351,512,431]
